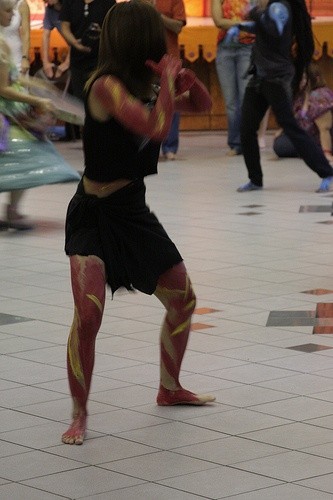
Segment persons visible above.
[0,0,333,232]
[59,0,217,446]
